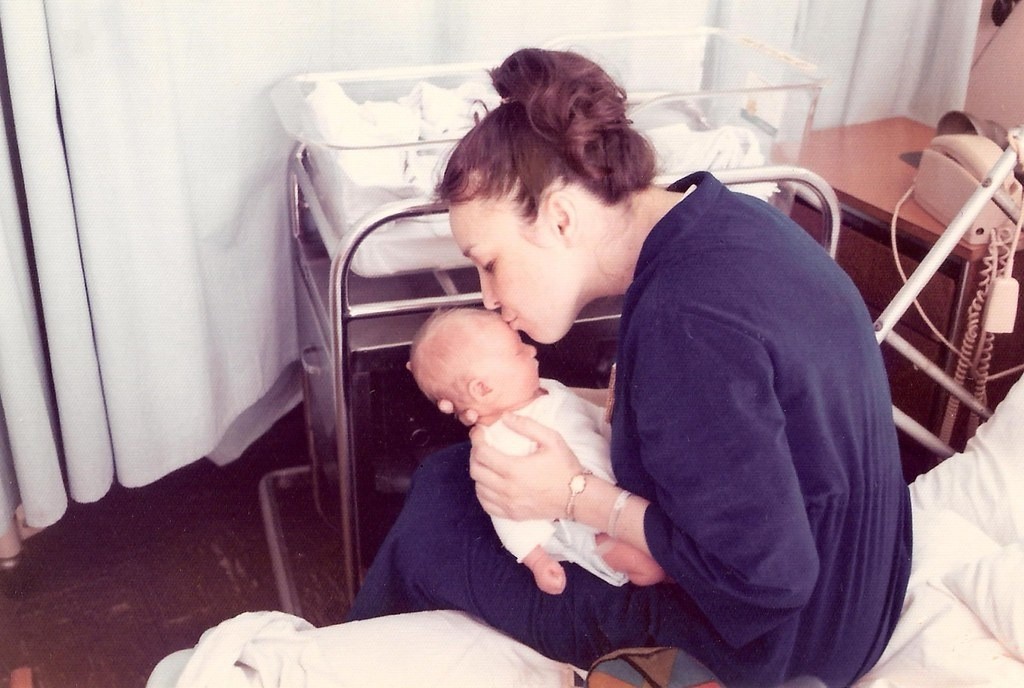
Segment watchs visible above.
[562,471,592,519]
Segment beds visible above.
[147,368,1024,688]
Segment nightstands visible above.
[770,116,1023,474]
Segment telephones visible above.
[915,133,1024,246]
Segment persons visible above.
[346,48,914,688]
[412,307,675,593]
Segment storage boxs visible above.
[269,29,831,281]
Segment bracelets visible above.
[605,489,631,539]
